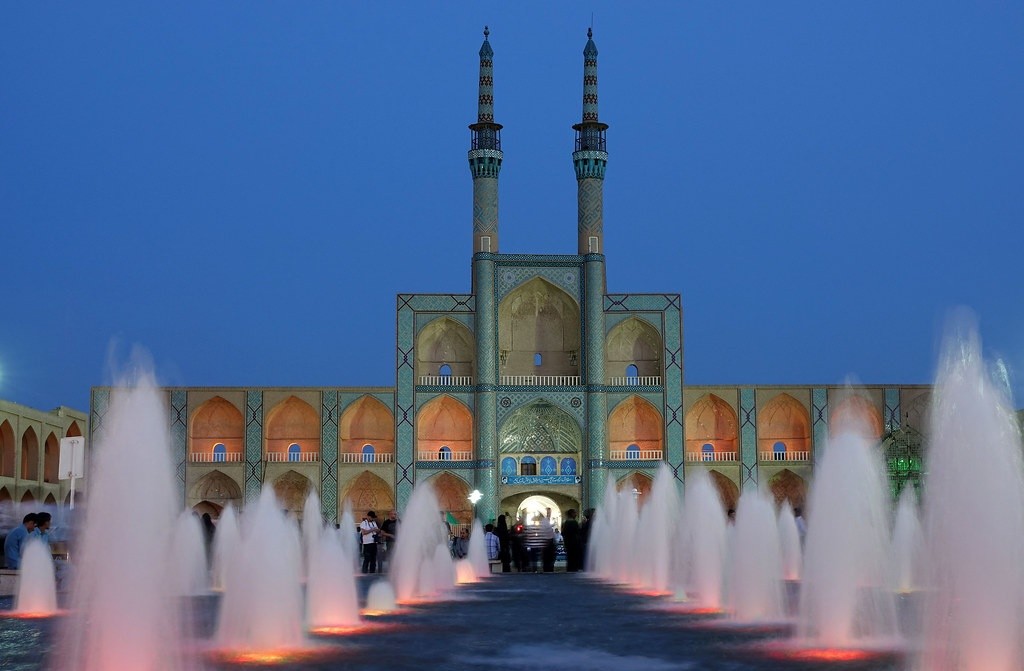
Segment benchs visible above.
[488,560,505,573]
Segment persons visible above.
[381,509,402,553]
[360,511,380,573]
[4,512,51,569]
[438,507,596,574]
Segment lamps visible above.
[570,351,578,366]
[500,350,507,367]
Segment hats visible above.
[367,511,377,518]
